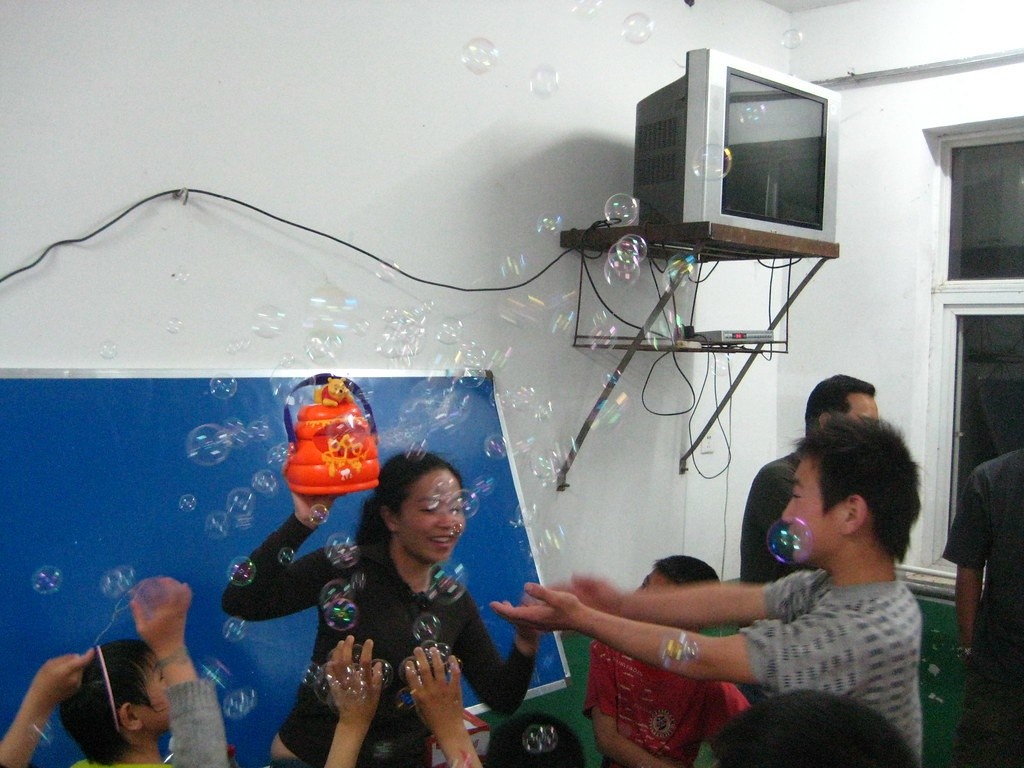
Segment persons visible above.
[0,374,925,767]
[943,449,1024,768]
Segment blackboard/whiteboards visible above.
[1,369,575,766]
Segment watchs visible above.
[956,647,973,661]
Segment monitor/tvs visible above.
[633,50,841,245]
